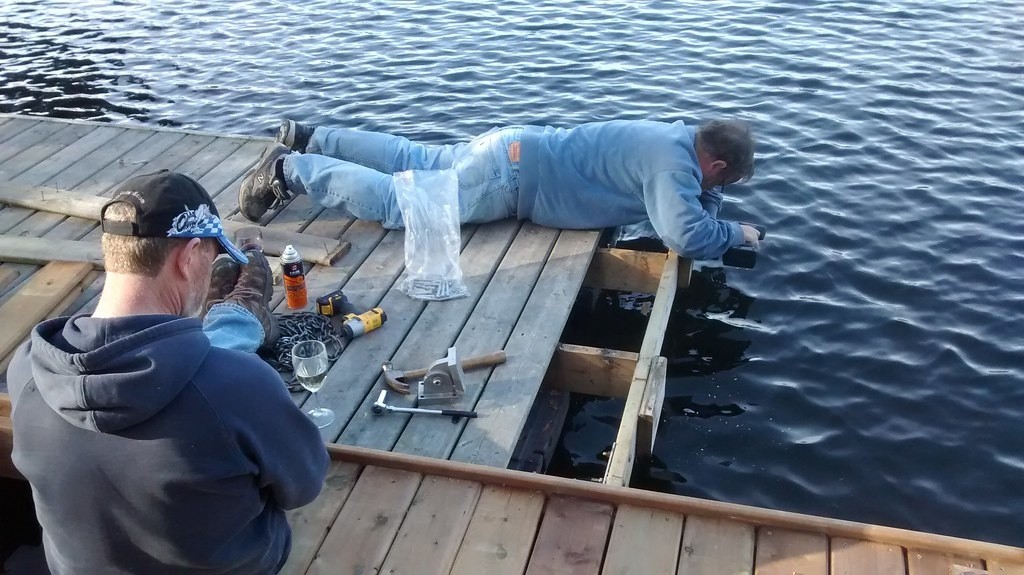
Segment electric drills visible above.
[722,220,767,269]
[313,290,389,345]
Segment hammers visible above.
[381,349,508,397]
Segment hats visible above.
[101,168,250,265]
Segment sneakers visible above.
[239,141,291,221]
[199,253,240,318]
[207,249,280,347]
[274,120,316,154]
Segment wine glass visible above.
[292,341,334,428]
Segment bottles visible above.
[280,245,308,309]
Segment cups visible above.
[234,226,264,256]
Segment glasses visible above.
[713,169,725,194]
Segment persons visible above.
[238,117,760,261]
[6,168,329,575]
